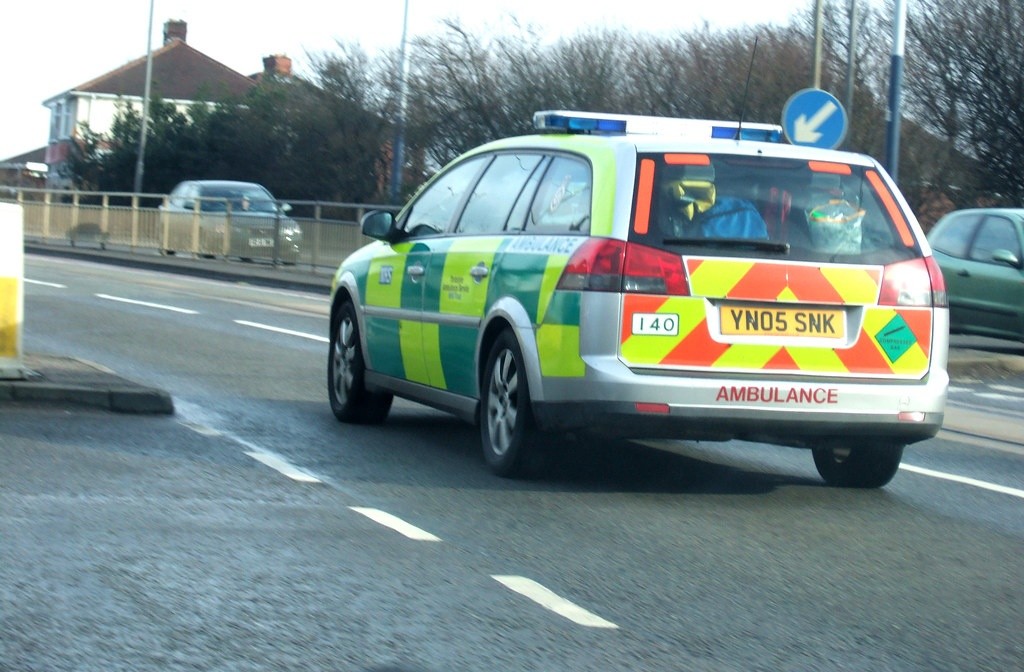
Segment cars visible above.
[156,180,302,264]
[926,208,1024,342]
[325,109,953,488]
